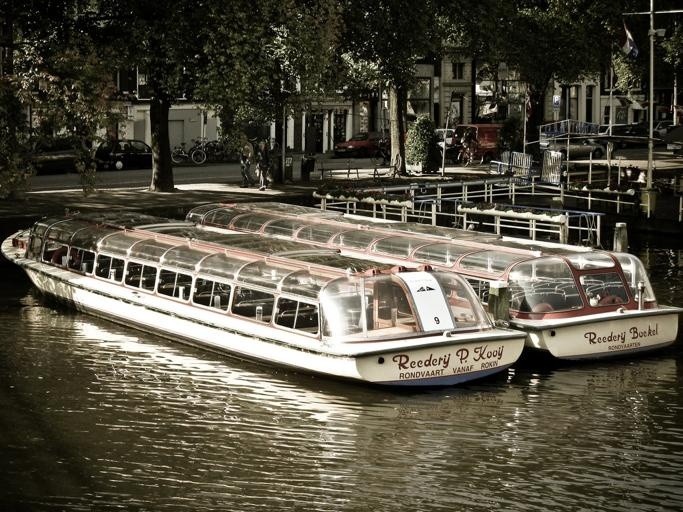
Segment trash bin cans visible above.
[272,154,293,184]
[302,157,315,173]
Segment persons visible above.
[255,140,269,192]
[238,143,254,186]
[451,127,479,158]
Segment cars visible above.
[540,119,683,160]
[0,136,91,176]
[94,140,152,170]
[334,132,389,158]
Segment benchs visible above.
[50,246,317,329]
[477,280,633,307]
[317,160,363,180]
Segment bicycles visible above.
[369,137,391,167]
[171,138,237,166]
[238,137,279,190]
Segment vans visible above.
[433,125,505,164]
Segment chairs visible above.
[373,154,401,184]
